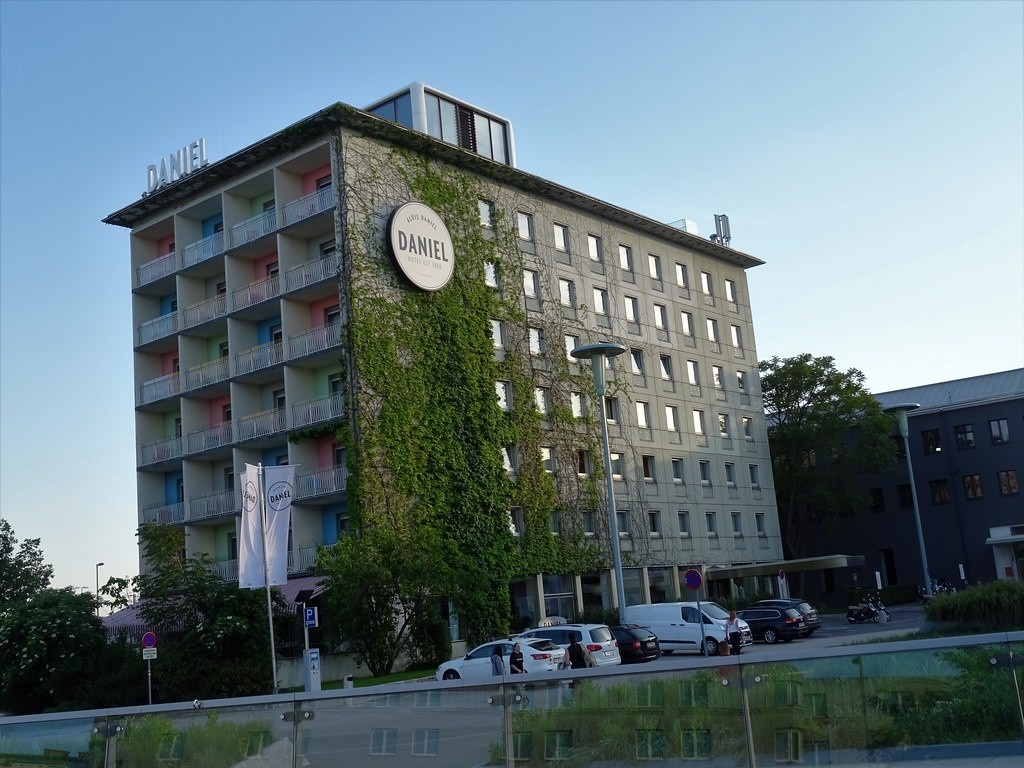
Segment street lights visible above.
[95,562,105,619]
[881,401,935,597]
[571,344,629,623]
[76,587,88,592]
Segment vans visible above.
[623,601,753,655]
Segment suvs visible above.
[728,598,822,645]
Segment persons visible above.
[491,644,507,676]
[719,641,732,655]
[565,633,587,670]
[726,611,744,655]
[510,642,527,675]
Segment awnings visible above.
[99,574,340,637]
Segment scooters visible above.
[916,578,957,608]
[846,591,891,625]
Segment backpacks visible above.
[580,643,597,668]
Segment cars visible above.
[436,637,566,680]
[606,622,662,665]
[494,624,621,670]
